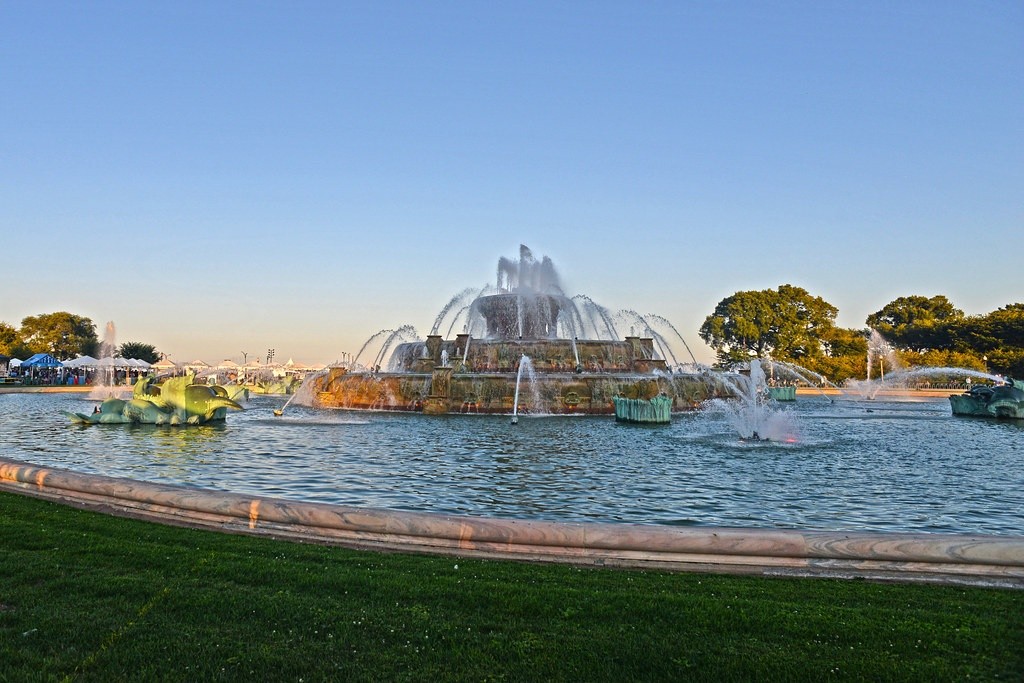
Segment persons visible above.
[994,374,1000,387]
[965,376,972,390]
[999,373,1005,386]
[766,373,853,389]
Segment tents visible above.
[0,353,330,387]
